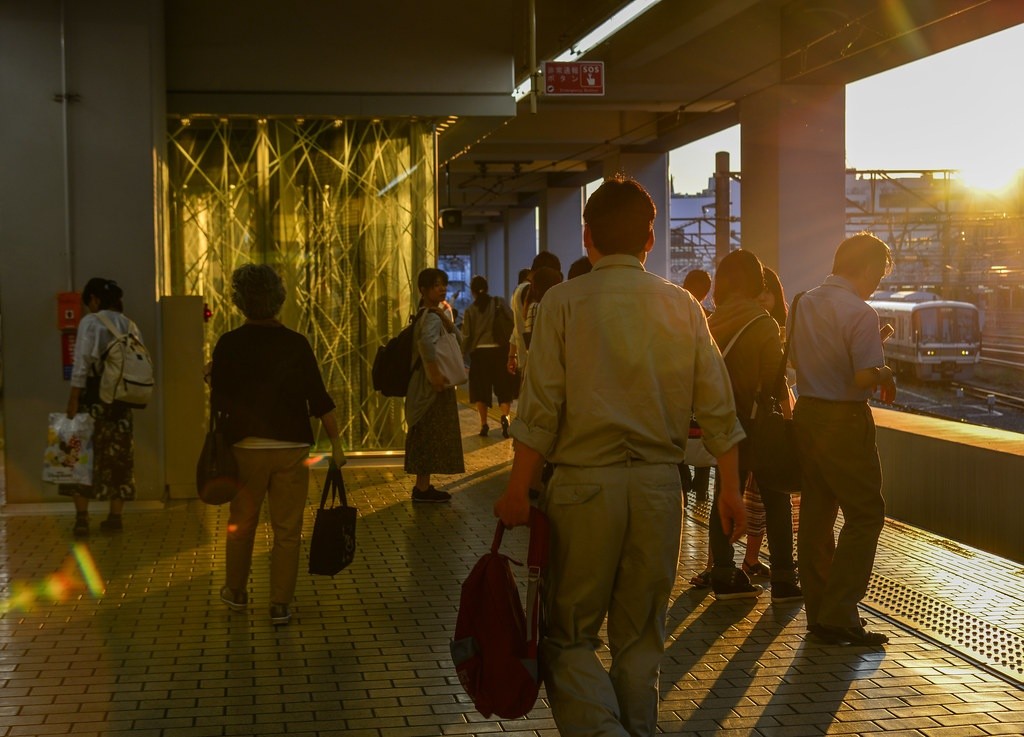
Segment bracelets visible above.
[203,371,212,383]
[508,353,518,358]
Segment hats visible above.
[535,266,561,296]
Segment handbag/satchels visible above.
[492,296,514,345]
[753,394,802,494]
[308,461,357,577]
[684,421,717,467]
[450,507,549,718]
[43,408,95,486]
[196,389,239,505]
[417,307,468,392]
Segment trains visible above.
[865,289,983,385]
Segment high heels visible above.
[500,415,509,438]
[478,424,489,437]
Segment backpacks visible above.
[92,312,155,409]
[372,307,444,397]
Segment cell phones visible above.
[879,324,894,342]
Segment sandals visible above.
[689,571,711,587]
[742,558,770,576]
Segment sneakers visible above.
[269,601,291,625]
[100,514,123,534]
[771,581,804,604]
[219,585,248,611]
[411,485,451,503]
[711,567,763,600]
[73,519,89,534]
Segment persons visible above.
[201,264,347,625]
[463,274,514,439]
[507,249,802,603]
[65,276,137,534]
[494,175,746,737]
[405,268,466,502]
[785,232,894,646]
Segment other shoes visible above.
[683,496,688,508]
[696,492,709,501]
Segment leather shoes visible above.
[807,618,889,648]
